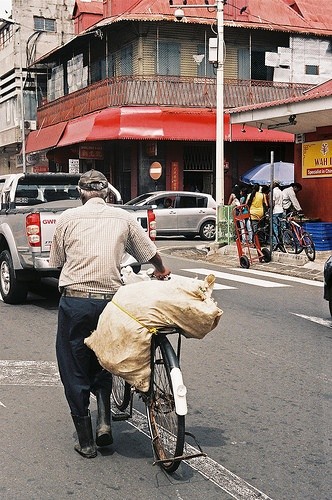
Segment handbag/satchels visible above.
[262,193,268,215]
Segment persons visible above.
[227,182,303,252]
[50,169,171,459]
[164,198,173,208]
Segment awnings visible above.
[230,123,294,142]
[55,105,232,147]
[18,121,58,156]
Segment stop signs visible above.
[149,161,162,181]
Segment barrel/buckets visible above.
[303,222,332,251]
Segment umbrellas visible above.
[240,160,294,186]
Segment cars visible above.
[122,190,216,241]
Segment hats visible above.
[78,169,108,190]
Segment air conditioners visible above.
[23,120,36,130]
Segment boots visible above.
[71,409,97,458]
[96,388,113,446]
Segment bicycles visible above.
[256,206,315,261]
[107,260,208,474]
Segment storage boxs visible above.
[303,222,332,250]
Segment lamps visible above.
[241,123,246,132]
[258,122,263,133]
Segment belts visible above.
[62,289,114,300]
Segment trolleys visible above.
[232,204,272,269]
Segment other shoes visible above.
[261,243,266,247]
[250,243,256,247]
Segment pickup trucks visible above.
[0,173,158,306]
